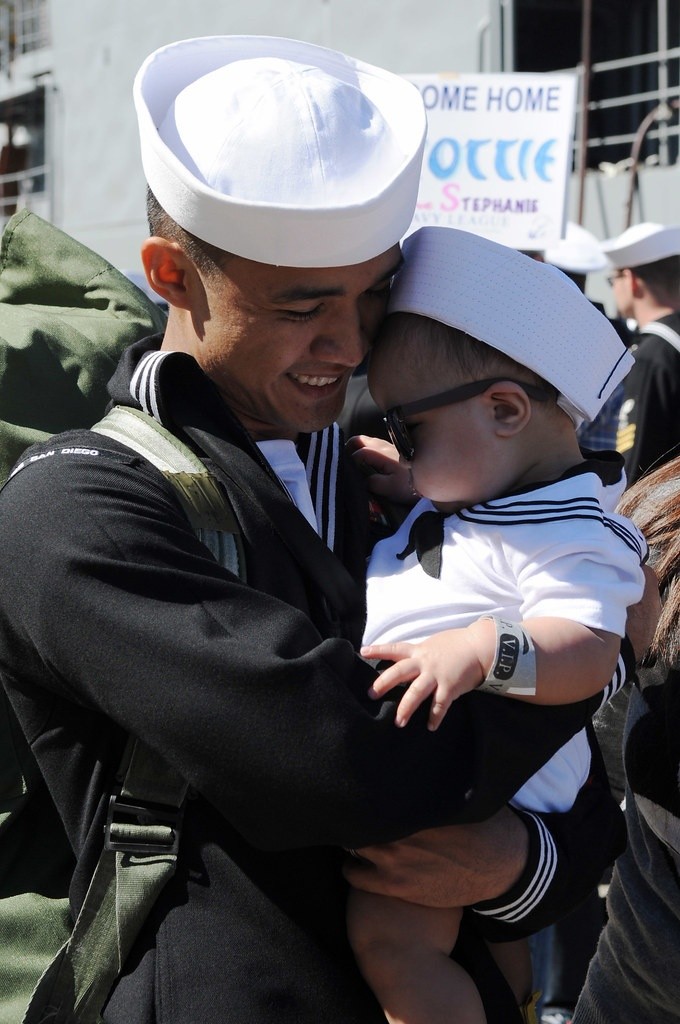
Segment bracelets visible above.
[473,611,523,696]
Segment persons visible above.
[2,34,680,1024]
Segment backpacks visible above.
[0,213,252,1024]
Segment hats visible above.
[602,221,680,269]
[132,34,428,267]
[386,226,636,432]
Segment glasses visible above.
[382,377,549,462]
